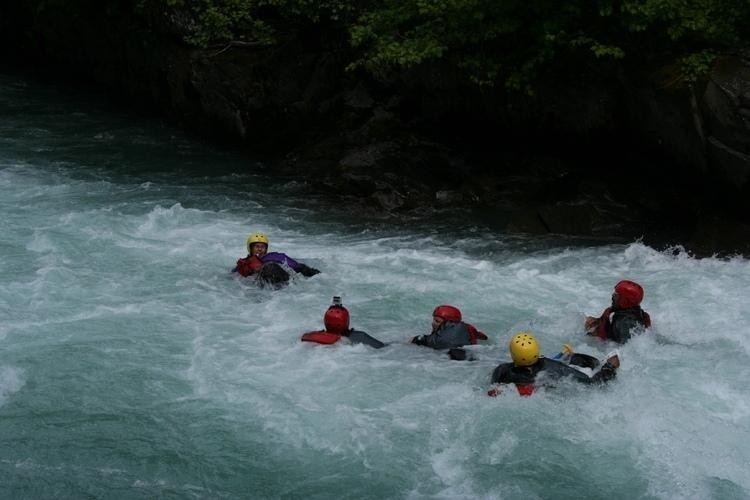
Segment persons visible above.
[411,305,488,361]
[231,233,321,291]
[301,305,385,349]
[585,279,650,345]
[488,333,620,397]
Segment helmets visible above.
[325,305,350,335]
[247,234,269,251]
[510,332,540,366]
[433,306,461,323]
[614,279,644,308]
